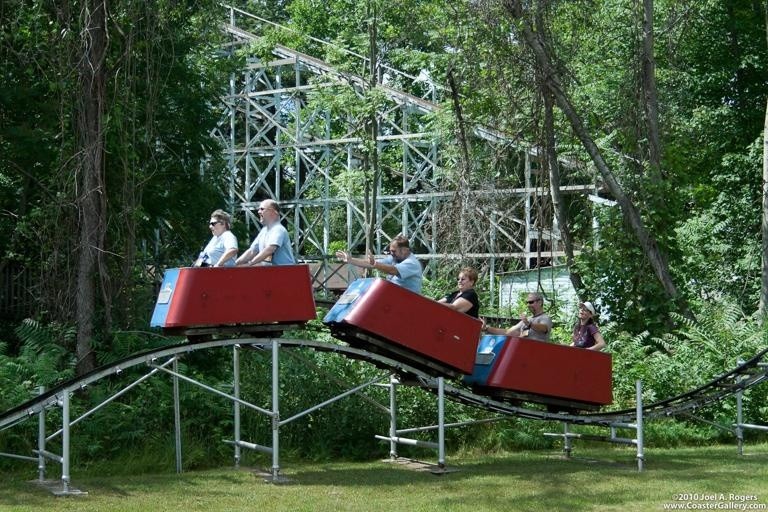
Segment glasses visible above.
[258,207,273,211]
[526,301,534,304]
[209,221,221,226]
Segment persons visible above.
[570,300,605,351]
[336,237,422,295]
[190,209,241,266]
[477,292,552,342]
[233,199,296,266]
[436,266,481,318]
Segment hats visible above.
[578,302,595,315]
